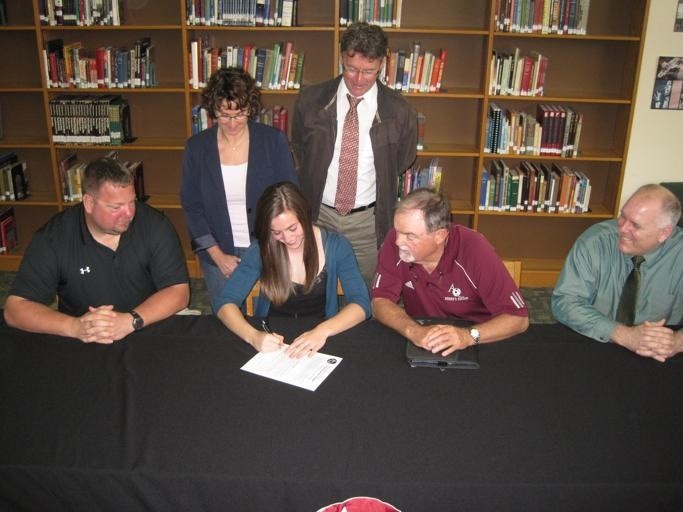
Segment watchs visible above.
[469,327,480,345]
[129,311,144,331]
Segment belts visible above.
[319,200,377,216]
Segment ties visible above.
[614,254,646,328]
[331,92,365,216]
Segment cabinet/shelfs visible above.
[0,0,651,292]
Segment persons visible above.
[370,188,529,356]
[211,181,370,360]
[292,21,418,289]
[180,67,298,316]
[3,157,191,345]
[550,183,683,363]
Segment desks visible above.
[0,315,683,512]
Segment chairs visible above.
[247,277,344,314]
[503,260,520,288]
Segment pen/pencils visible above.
[262,320,274,334]
